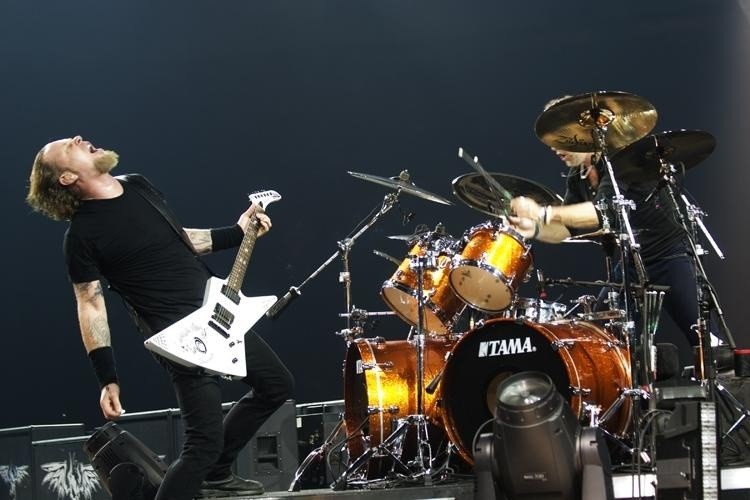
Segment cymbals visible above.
[451,172,563,217]
[535,91,657,152]
[599,127,714,179]
[348,170,454,206]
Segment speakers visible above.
[0,421,85,500]
[111,398,298,491]
[33,434,113,500]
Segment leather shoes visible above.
[197,473,265,497]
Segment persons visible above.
[24,134,296,500]
[497,93,715,349]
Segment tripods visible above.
[288,123,750,490]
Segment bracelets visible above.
[87,346,119,387]
[210,224,245,253]
[530,222,540,241]
[544,204,552,228]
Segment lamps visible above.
[82,421,165,500]
[472,370,614,499]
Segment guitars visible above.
[145,190,282,382]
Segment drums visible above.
[440,318,632,465]
[380,231,468,337]
[343,334,465,479]
[447,223,533,313]
